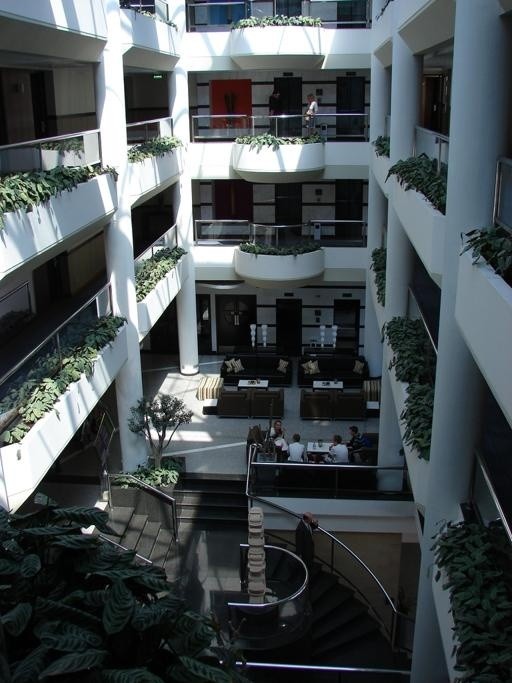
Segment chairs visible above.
[334,390,365,421]
[217,388,251,419]
[300,389,334,421]
[251,388,284,419]
[247,425,379,499]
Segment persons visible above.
[264,419,370,464]
[295,510,319,590]
[267,88,290,135]
[222,88,238,124]
[302,91,319,137]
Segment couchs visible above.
[221,358,292,387]
[298,357,369,387]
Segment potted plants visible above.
[256,437,277,481]
